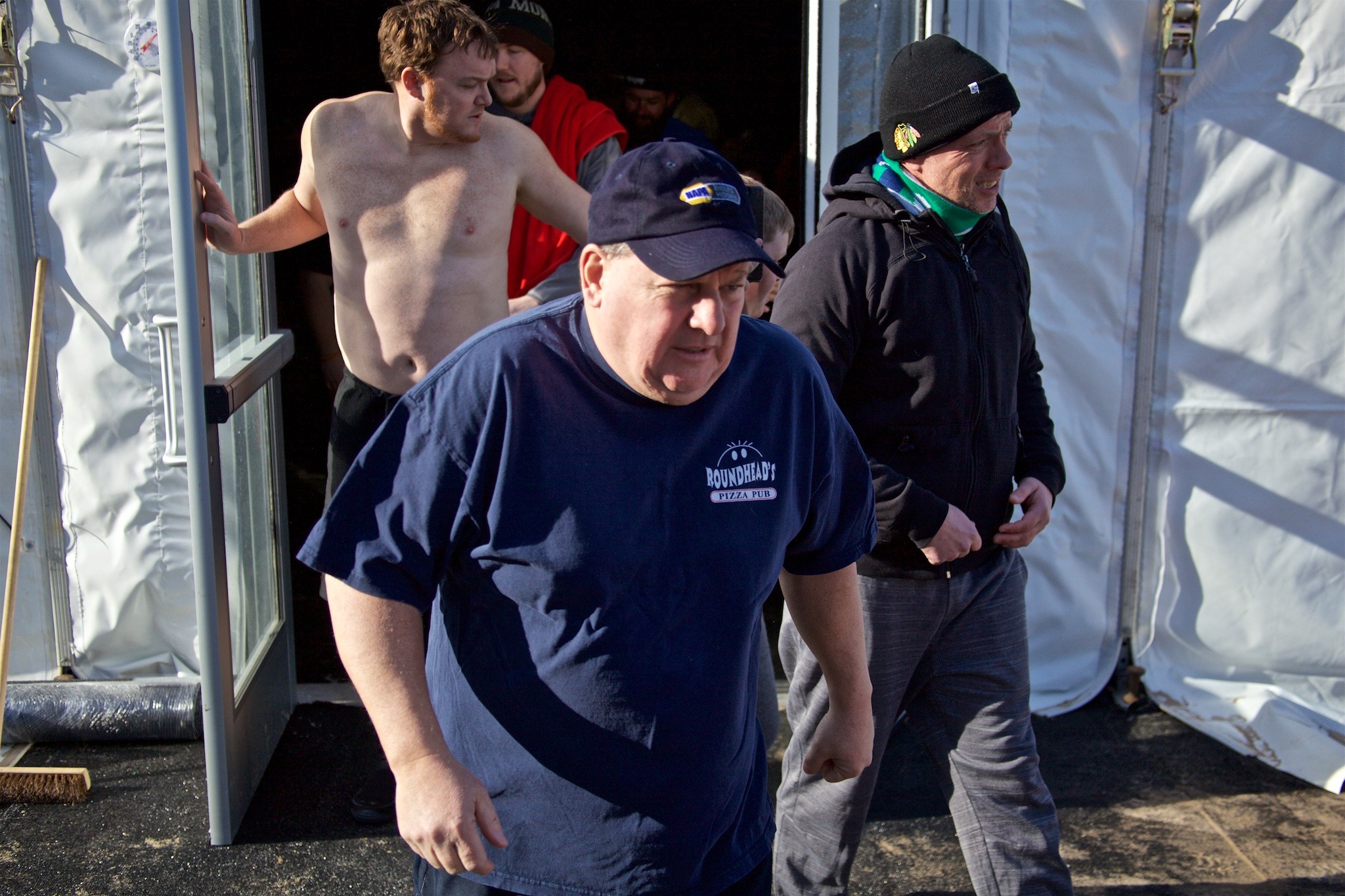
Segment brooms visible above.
[0,256,94,810]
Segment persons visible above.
[295,137,876,896]
[769,34,1073,896]
[484,0,628,317]
[618,55,715,155]
[194,0,594,512]
[738,174,795,317]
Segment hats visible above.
[880,34,1022,162]
[624,58,682,92]
[586,137,787,281]
[484,1,553,72]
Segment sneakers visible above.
[351,758,393,823]
